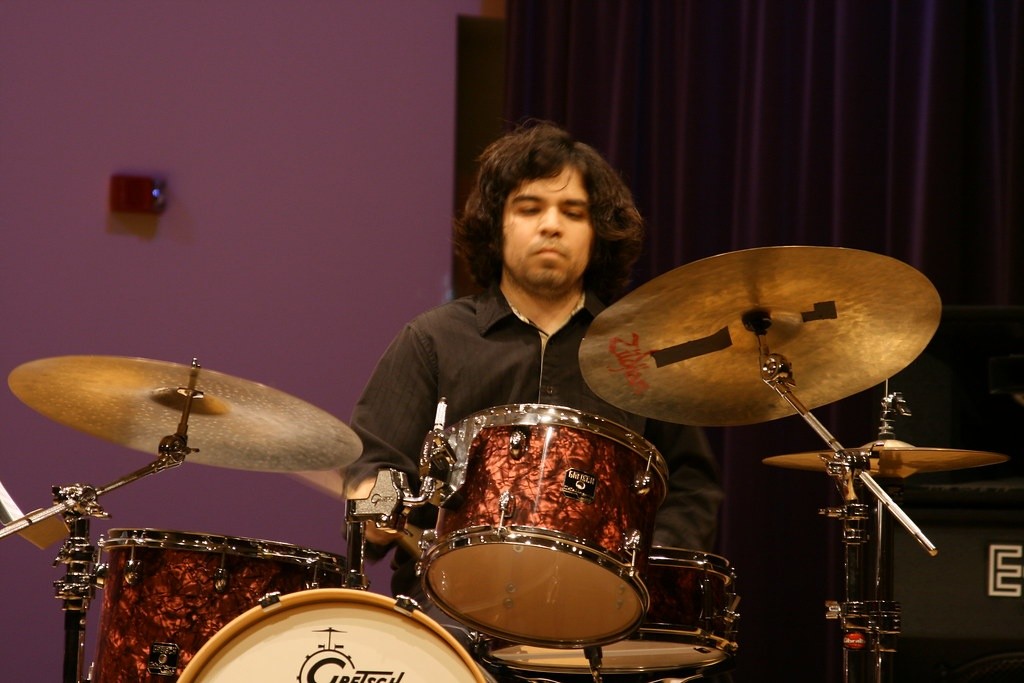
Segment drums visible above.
[414,404,670,650]
[466,545,741,675]
[87,528,347,683]
[175,588,488,683]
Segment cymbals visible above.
[762,439,1011,479]
[7,355,364,473]
[578,246,942,427]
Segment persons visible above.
[341,122,724,660]
